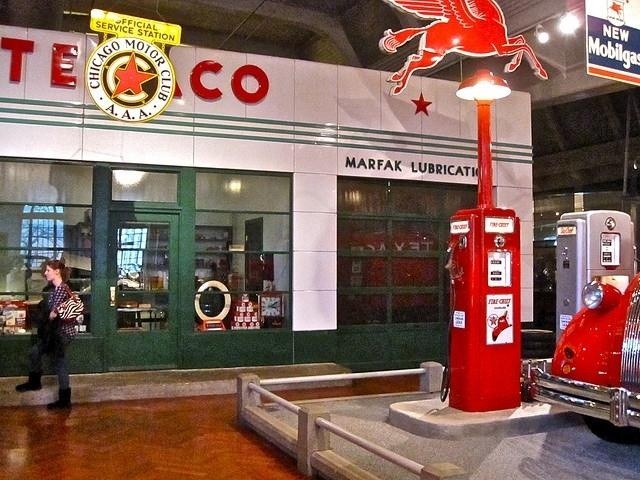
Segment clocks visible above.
[260,296,282,317]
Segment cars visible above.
[118,255,273,304]
[525,269,640,447]
[19,278,150,330]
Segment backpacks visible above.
[56,295,85,324]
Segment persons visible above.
[14,259,79,412]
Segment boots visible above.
[46,387,72,411]
[15,368,43,393]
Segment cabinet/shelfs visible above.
[145,222,233,293]
